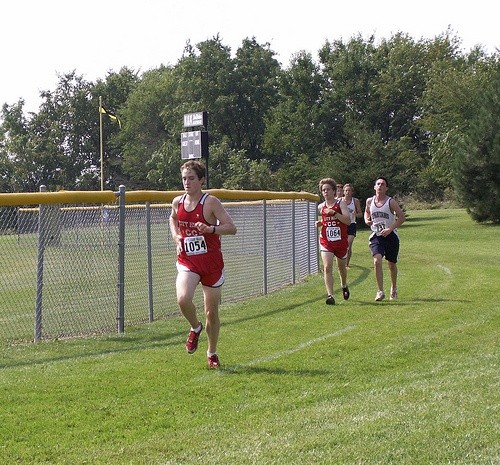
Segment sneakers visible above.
[389,289,398,300]
[342,285,350,300]
[325,295,335,305]
[207,354,221,369]
[375,290,385,302]
[185,320,202,354]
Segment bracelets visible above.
[332,211,337,216]
[390,226,395,231]
[212,227,215,234]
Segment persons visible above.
[338,184,362,270]
[169,160,237,368]
[334,184,344,200]
[314,178,352,305]
[364,177,407,301]
